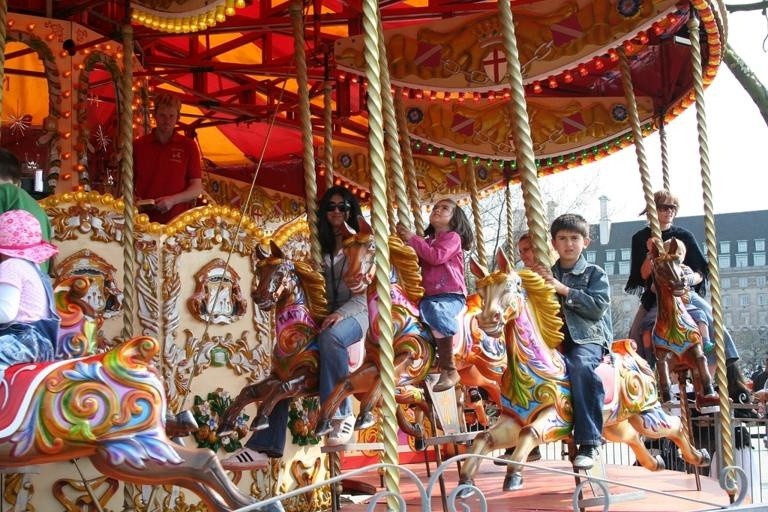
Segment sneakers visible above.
[325,414,357,446]
[221,446,270,471]
[573,444,600,469]
[354,410,378,430]
[503,472,526,492]
[414,422,428,452]
[493,445,542,466]
[314,419,335,436]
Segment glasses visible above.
[325,203,350,212]
[657,204,676,212]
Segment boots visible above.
[432,336,461,392]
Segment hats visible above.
[0,208,59,265]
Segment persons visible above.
[634,358,768,503]
[494,234,553,466]
[0,149,51,274]
[625,191,739,368]
[219,186,370,469]
[395,198,473,392]
[494,214,615,470]
[0,209,59,389]
[132,92,202,224]
[640,238,715,371]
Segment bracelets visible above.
[645,252,654,261]
